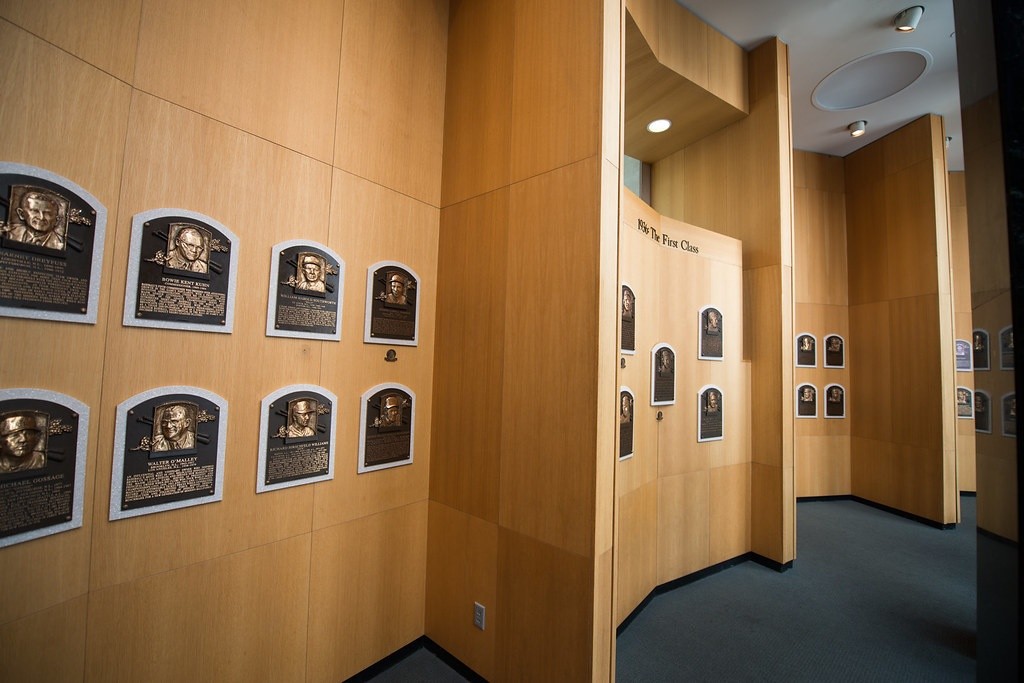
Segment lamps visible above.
[895,6,924,33]
[848,120,866,138]
